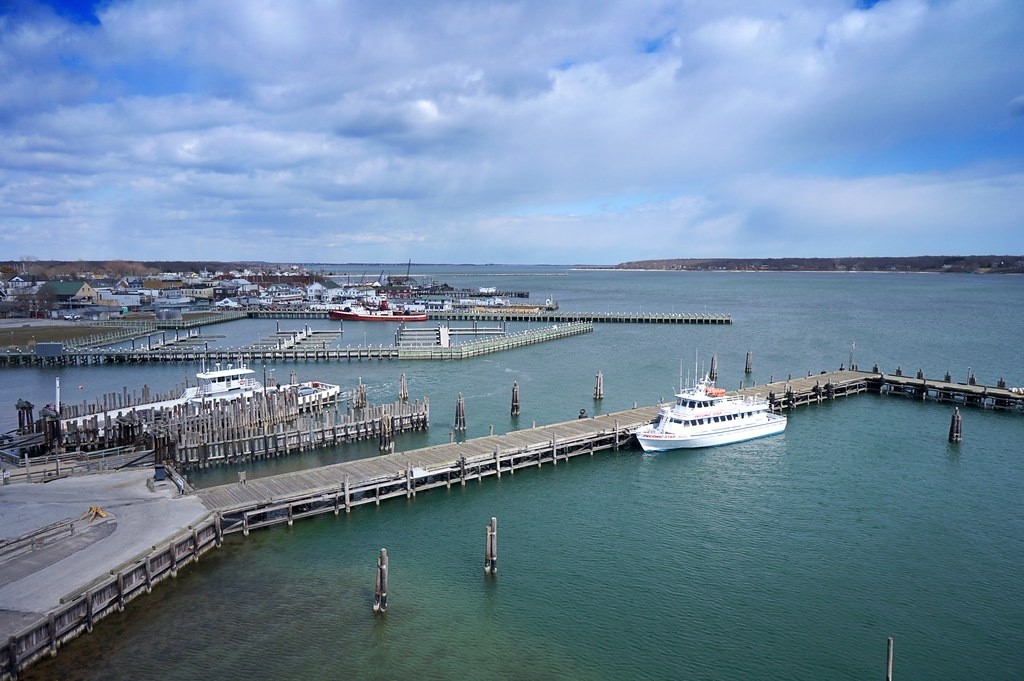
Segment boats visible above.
[630,346,788,454]
[326,304,427,323]
[54,356,341,443]
